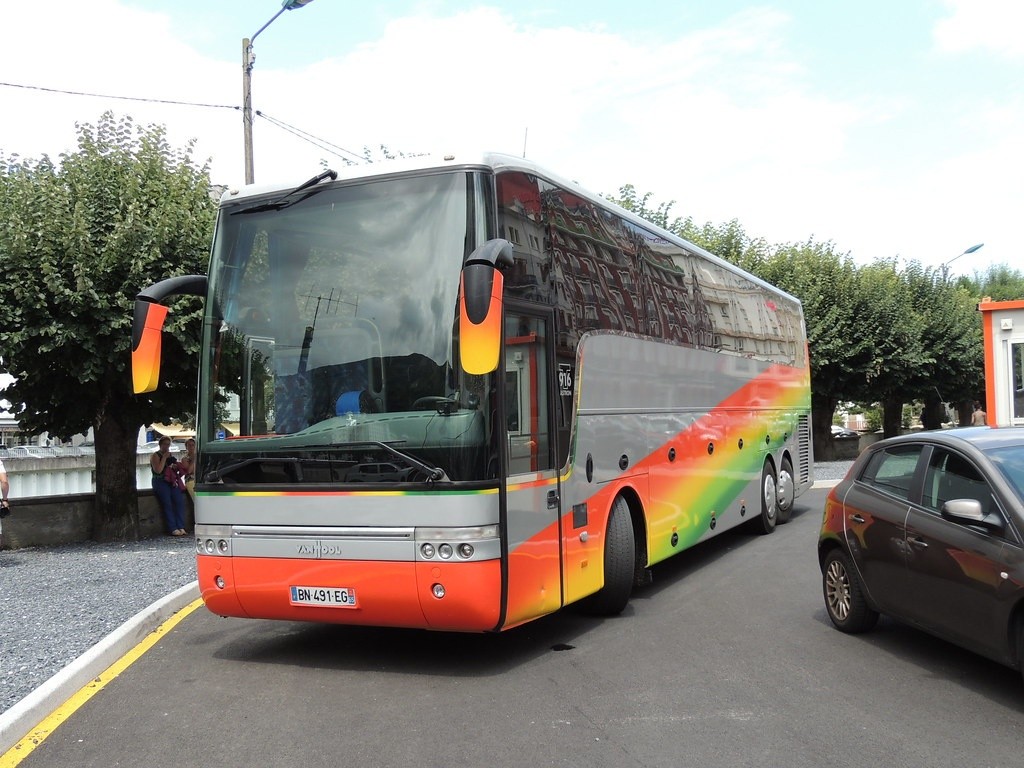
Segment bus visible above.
[129,148,814,640]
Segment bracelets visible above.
[2,499,8,502]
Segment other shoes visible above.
[181,529,187,536]
[169,530,183,536]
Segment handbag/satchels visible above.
[164,468,177,483]
[0,499,10,518]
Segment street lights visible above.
[244,0,313,185]
[943,243,988,272]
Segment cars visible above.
[816,428,1024,697]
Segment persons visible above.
[970,402,988,427]
[918,400,949,431]
[150,436,189,537]
[0,456,11,537]
[181,438,195,505]
